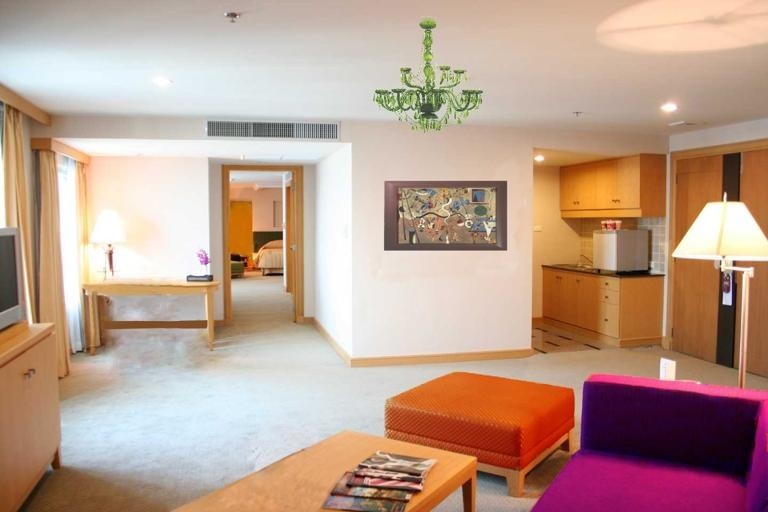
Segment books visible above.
[356,447,436,473]
[330,470,415,501]
[322,493,406,512]
[346,474,424,492]
[354,465,427,482]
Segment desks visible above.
[80,270,222,355]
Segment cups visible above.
[600,219,622,231]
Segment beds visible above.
[254,232,283,275]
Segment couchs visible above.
[527,373,768,511]
[231,251,246,278]
[382,369,575,497]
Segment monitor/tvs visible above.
[0,228,23,332]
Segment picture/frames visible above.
[383,179,508,251]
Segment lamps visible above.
[373,17,483,134]
[670,192,768,387]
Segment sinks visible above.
[570,265,590,270]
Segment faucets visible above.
[580,254,593,264]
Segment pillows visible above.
[262,239,283,250]
[231,251,241,261]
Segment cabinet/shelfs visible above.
[597,154,666,217]
[542,265,560,334]
[734,138,767,376]
[670,141,740,369]
[560,269,595,332]
[559,162,597,219]
[595,266,666,349]
[0,323,62,512]
